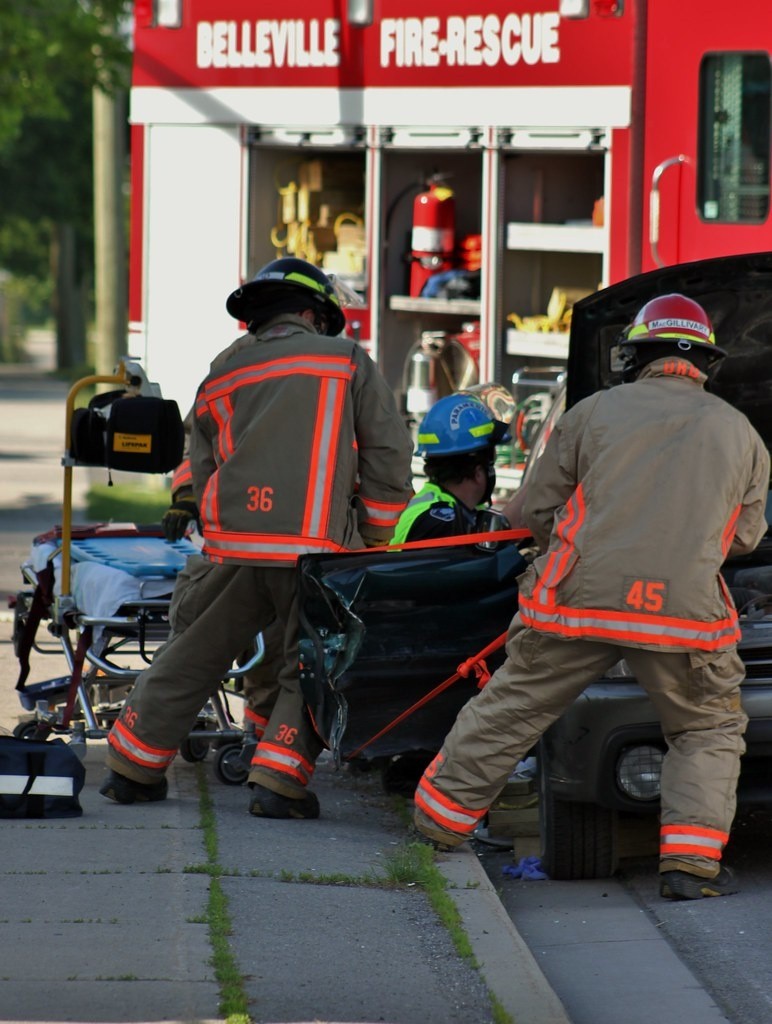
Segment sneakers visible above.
[659,866,742,898]
[414,807,466,851]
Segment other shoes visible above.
[499,782,541,807]
[99,771,169,803]
[249,784,325,820]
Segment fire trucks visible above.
[126,1,771,515]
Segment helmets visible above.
[412,394,513,456]
[620,293,728,357]
[227,257,347,338]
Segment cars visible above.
[297,252,772,878]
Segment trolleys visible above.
[20,359,272,787]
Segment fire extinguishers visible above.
[453,322,480,374]
[410,172,457,298]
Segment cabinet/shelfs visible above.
[254,127,614,479]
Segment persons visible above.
[99,258,414,817]
[388,393,508,553]
[411,295,772,897]
